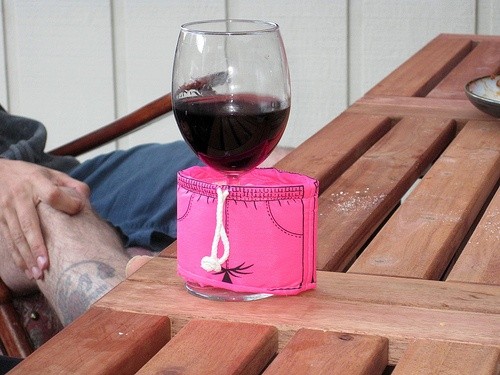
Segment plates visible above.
[464,74,499,118]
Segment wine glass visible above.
[170,19,292,301]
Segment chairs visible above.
[1,68,229,359]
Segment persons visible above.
[0,104,297,329]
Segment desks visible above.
[4,33,500,375]
